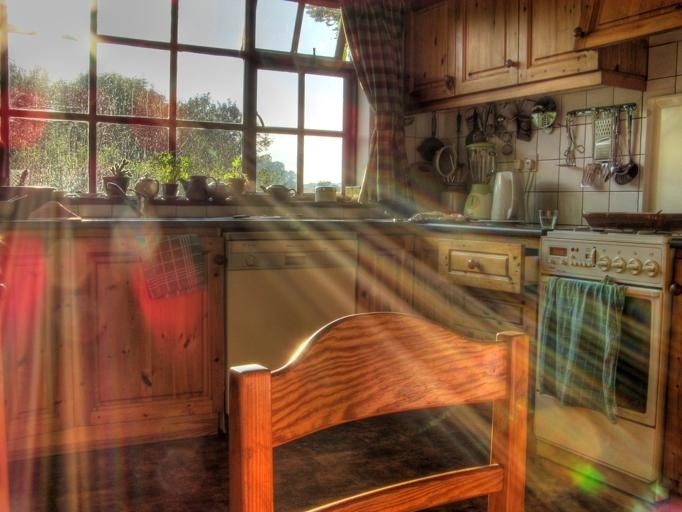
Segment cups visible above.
[315,186,337,201]
[537,208,558,230]
[344,185,361,204]
[0,201,17,219]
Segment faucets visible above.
[106,182,150,219]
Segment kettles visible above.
[491,170,520,221]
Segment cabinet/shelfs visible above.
[72,228,224,451]
[662,250,682,498]
[405,0,456,110]
[456,1,648,107]
[415,232,538,404]
[0,230,76,461]
[356,226,414,317]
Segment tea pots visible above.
[178,175,219,200]
[209,178,233,201]
[260,183,298,203]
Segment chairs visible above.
[227,308,532,512]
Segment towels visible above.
[534,274,626,426]
[407,210,467,224]
[133,233,209,301]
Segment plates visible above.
[466,219,522,226]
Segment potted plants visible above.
[102,158,134,197]
[150,149,193,199]
[223,155,249,194]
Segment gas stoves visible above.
[540,228,682,288]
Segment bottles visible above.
[440,184,467,215]
[135,174,160,199]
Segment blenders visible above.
[462,143,504,220]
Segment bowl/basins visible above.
[0,186,54,220]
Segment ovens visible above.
[531,271,675,504]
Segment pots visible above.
[417,112,445,164]
